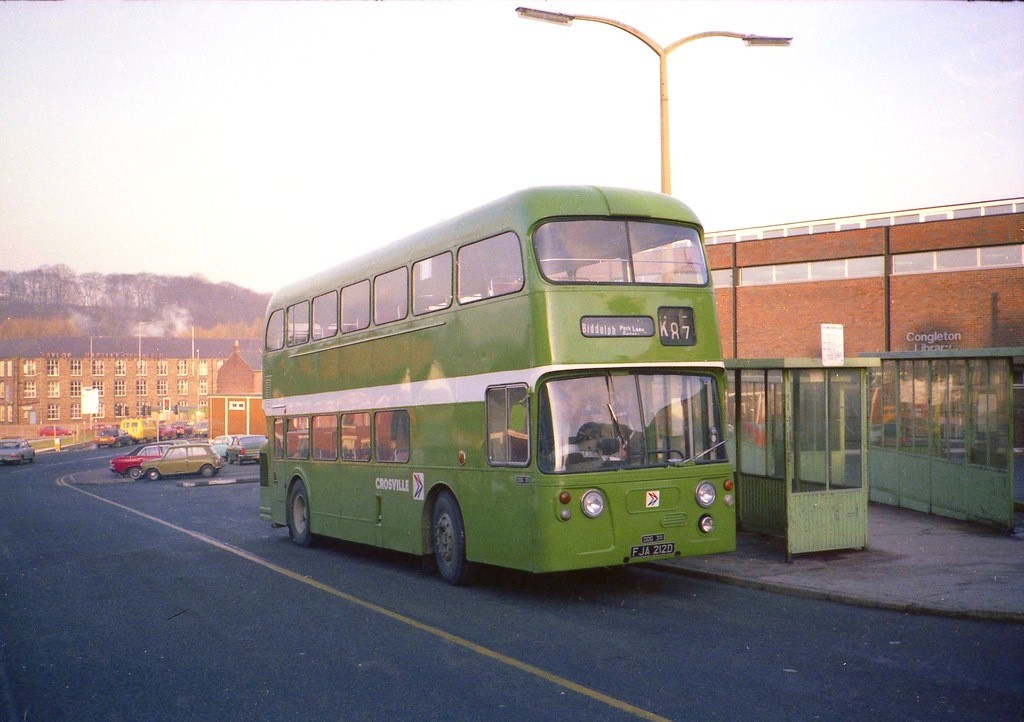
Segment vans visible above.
[119,418,159,443]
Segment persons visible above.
[541,394,626,464]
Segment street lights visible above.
[516,3,793,195]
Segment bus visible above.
[258,184,737,587]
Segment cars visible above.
[115,445,177,479]
[37,425,71,436]
[109,442,176,473]
[225,435,267,466]
[171,422,193,439]
[0,439,35,465]
[159,424,177,440]
[94,429,134,449]
[140,444,223,481]
[193,420,209,437]
[212,436,239,460]
